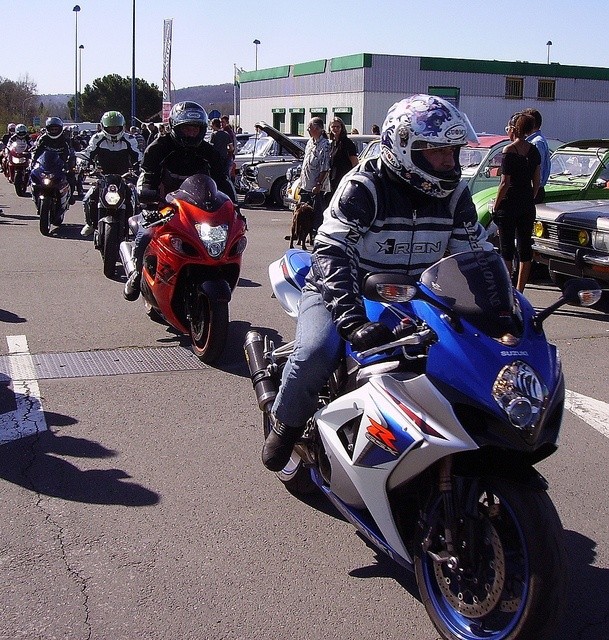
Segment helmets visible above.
[380,93,478,199]
[45,117,64,139]
[8,123,16,135]
[100,111,126,142]
[15,123,27,139]
[168,101,208,148]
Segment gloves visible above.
[141,209,163,223]
[76,162,88,173]
[340,317,395,356]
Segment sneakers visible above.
[80,223,94,237]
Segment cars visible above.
[230,131,380,211]
[527,199,608,290]
[460,133,568,196]
[467,138,609,246]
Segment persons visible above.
[28,125,46,153]
[254,121,268,138]
[5,124,35,182]
[370,124,381,136]
[221,114,237,180]
[328,117,359,201]
[490,112,541,294]
[28,117,76,215]
[62,124,102,152]
[261,95,499,471]
[283,117,331,245]
[123,102,240,301]
[128,122,169,152]
[76,110,143,237]
[209,118,234,177]
[0,123,17,173]
[350,128,359,135]
[231,154,237,185]
[521,107,551,205]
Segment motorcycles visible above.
[119,173,247,361]
[25,147,75,235]
[73,150,143,279]
[243,247,604,640]
[0,139,34,197]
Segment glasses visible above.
[50,128,59,131]
[331,125,342,128]
[505,126,516,133]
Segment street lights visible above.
[73,5,81,123]
[253,39,261,71]
[79,45,84,93]
[546,40,552,64]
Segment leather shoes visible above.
[261,418,306,471]
[123,270,141,301]
